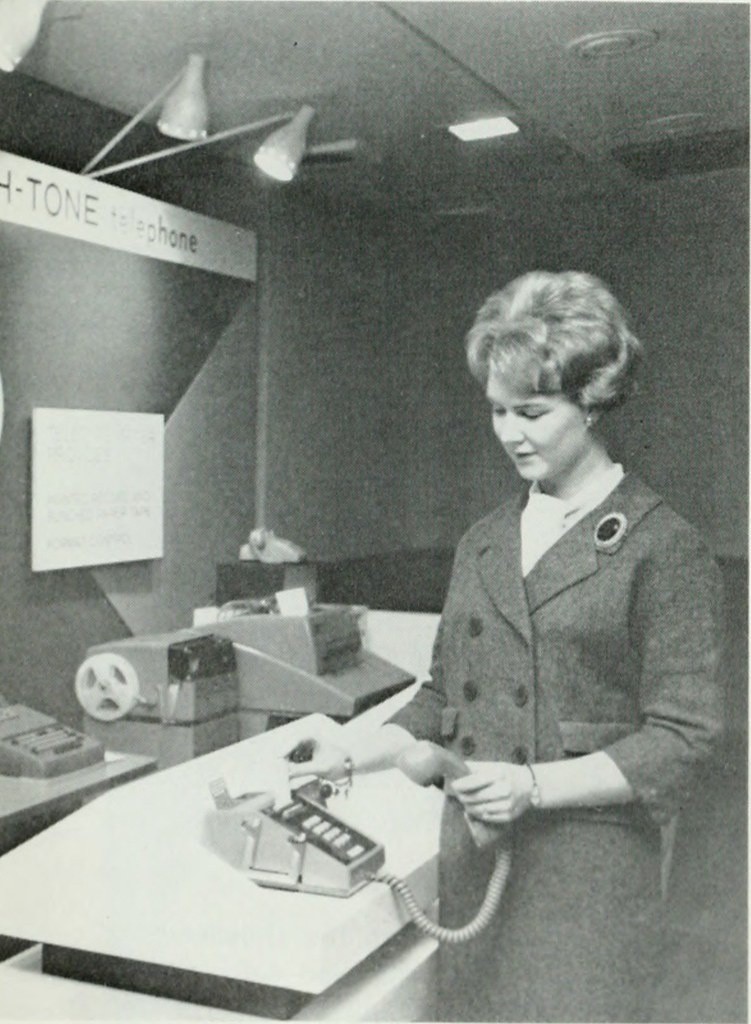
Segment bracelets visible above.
[333,745,358,796]
[528,765,543,814]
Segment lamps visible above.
[80,55,211,173]
[88,100,316,184]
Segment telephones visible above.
[397,741,514,849]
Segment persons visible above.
[260,265,729,1023]
